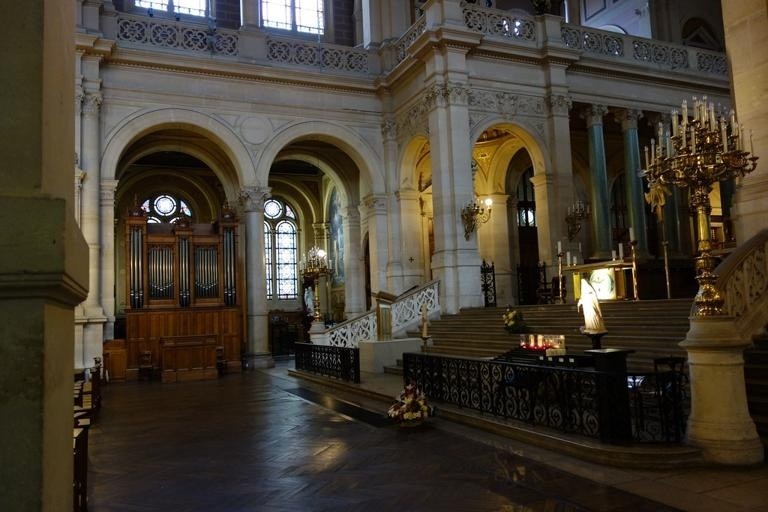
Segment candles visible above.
[557,226,636,268]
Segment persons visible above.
[577,279,605,333]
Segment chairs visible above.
[636,356,686,439]
[534,275,567,306]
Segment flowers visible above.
[386,380,439,422]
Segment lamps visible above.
[564,201,592,240]
[638,92,758,317]
[458,198,494,240]
[297,245,335,321]
